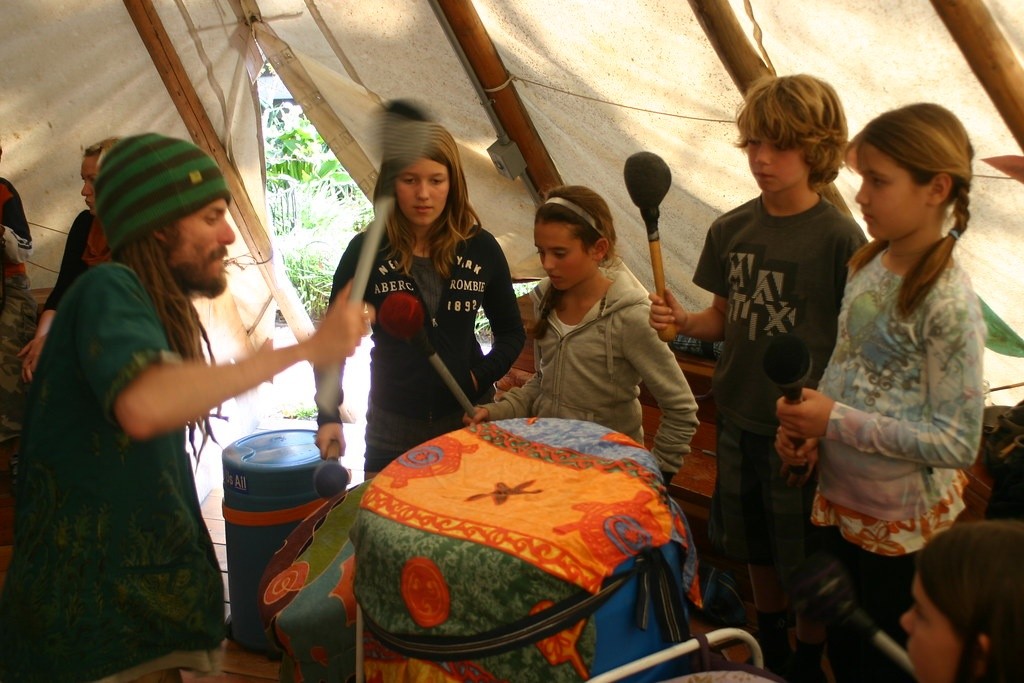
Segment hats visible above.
[94,133,231,251]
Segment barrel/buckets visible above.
[221,430,345,645]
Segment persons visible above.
[898,520,1023,683]
[0,133,375,683]
[775,102,986,682]
[315,122,527,481]
[649,72,868,682]
[464,184,701,487]
